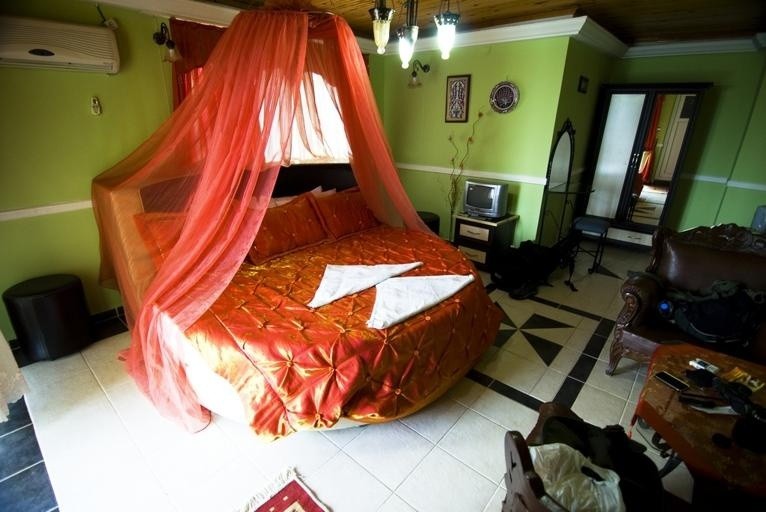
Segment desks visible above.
[627,344,766,496]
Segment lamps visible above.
[369,0,460,88]
[153,23,183,62]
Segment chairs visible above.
[502,402,663,512]
[625,172,643,222]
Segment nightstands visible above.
[3,273,97,360]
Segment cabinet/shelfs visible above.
[452,211,519,270]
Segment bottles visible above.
[658,299,674,319]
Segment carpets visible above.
[236,467,333,512]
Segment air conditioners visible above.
[0,15,120,73]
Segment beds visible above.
[91,162,504,432]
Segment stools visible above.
[567,216,612,274]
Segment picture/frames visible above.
[445,73,470,123]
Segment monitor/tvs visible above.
[463,180,508,219]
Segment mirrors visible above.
[538,118,576,248]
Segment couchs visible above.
[605,222,766,377]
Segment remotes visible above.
[689,358,719,374]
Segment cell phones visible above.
[654,370,689,393]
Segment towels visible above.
[306,261,475,330]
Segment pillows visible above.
[132,186,380,276]
